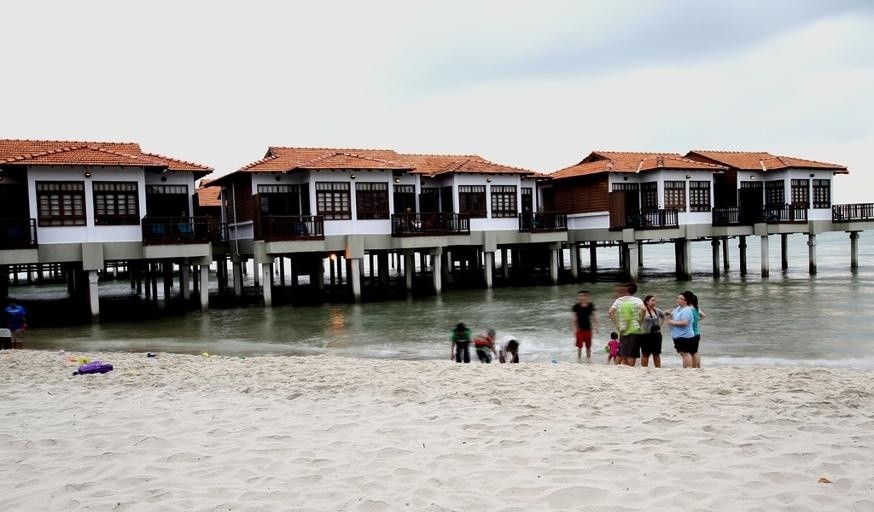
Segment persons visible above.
[571,291,598,363]
[606,284,706,368]
[473,327,497,362]
[499,336,519,364]
[450,322,472,363]
[5,303,27,348]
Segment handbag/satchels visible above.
[78,362,112,373]
